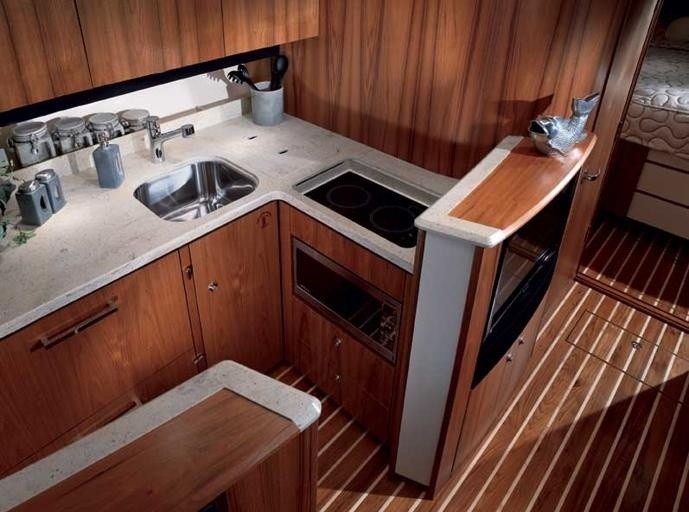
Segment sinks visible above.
[134,154,259,223]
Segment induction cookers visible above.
[292,158,440,252]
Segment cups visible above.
[249,79,285,126]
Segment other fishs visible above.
[528,91,603,159]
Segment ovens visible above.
[286,235,403,368]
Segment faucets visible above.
[145,115,196,164]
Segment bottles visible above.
[14,168,66,228]
[8,108,152,170]
